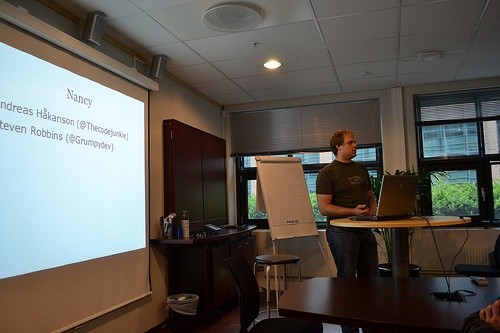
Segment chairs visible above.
[223,252,323,333]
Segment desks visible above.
[278,277,500,333]
[330,214,472,278]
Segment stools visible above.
[253,253,301,319]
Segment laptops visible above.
[348,175,418,221]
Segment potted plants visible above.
[370,169,451,278]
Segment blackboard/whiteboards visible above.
[256,157,318,240]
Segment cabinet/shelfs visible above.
[149,119,257,333]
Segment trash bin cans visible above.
[167,294,199,333]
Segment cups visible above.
[181,219,189,238]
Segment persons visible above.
[315,130,379,333]
[462,297,500,333]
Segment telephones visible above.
[204,223,223,232]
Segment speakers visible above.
[82,13,106,46]
[149,55,169,81]
